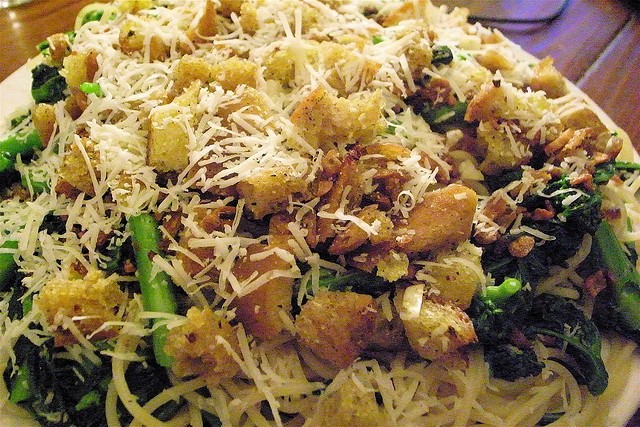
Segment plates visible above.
[1,13,640,427]
